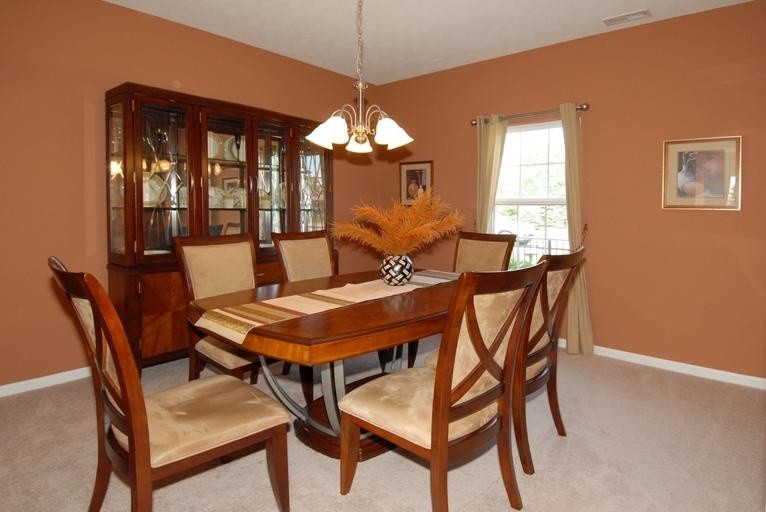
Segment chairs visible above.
[514,245,587,477]
[45,253,294,511]
[224,222,240,234]
[269,230,393,368]
[339,260,531,512]
[407,227,518,369]
[172,234,290,388]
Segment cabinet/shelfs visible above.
[289,114,333,238]
[201,93,289,292]
[105,81,201,378]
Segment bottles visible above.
[678,152,700,193]
[408,180,423,198]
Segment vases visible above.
[380,253,415,285]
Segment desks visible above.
[187,266,467,466]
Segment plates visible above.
[120,173,168,207]
[208,132,247,208]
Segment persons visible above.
[408,177,419,200]
[677,152,722,197]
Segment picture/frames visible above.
[397,158,435,209]
[661,135,747,217]
[221,177,240,197]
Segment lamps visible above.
[206,161,223,178]
[305,3,415,158]
[150,156,179,173]
